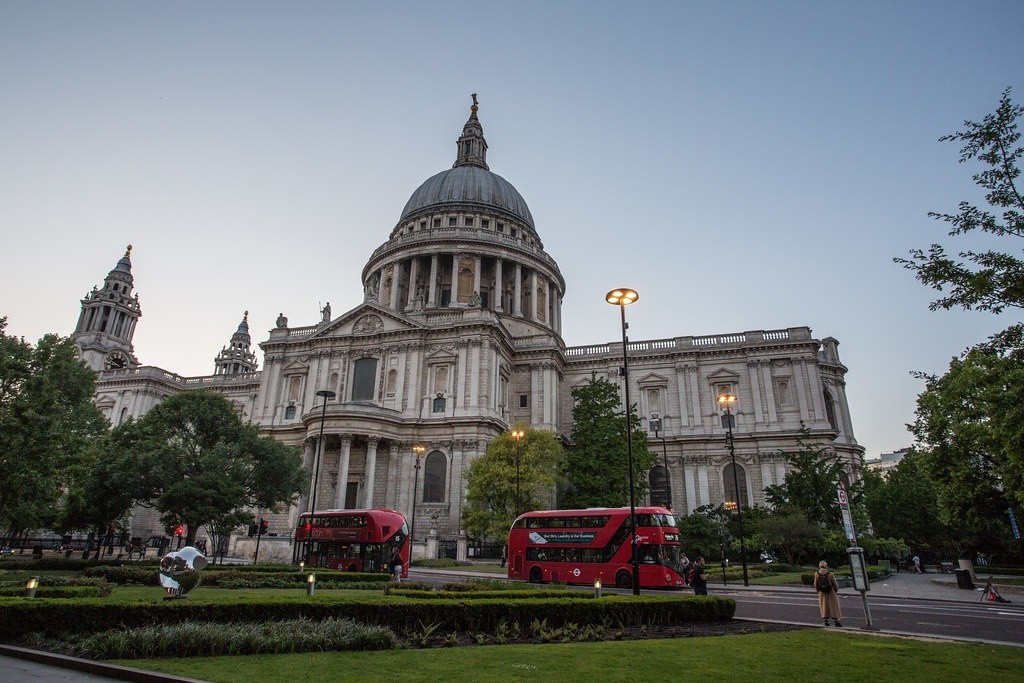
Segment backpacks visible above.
[816,571,830,592]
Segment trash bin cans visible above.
[955,569,972,589]
[940,562,953,573]
[33,545,42,559]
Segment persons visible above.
[501,517,707,594]
[53,542,147,561]
[912,554,922,574]
[814,561,843,627]
[301,517,403,574]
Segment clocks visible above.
[105,352,129,370]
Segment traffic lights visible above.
[260,519,268,535]
[253,524,258,534]
[176,526,183,538]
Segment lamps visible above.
[593,578,602,588]
[26,575,40,589]
[307,572,316,583]
[393,565,403,574]
[299,560,305,566]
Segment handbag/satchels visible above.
[684,557,693,570]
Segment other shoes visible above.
[835,621,842,627]
[824,620,830,626]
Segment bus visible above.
[507,506,687,591]
[291,508,411,578]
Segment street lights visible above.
[305,390,336,566]
[409,446,425,568]
[512,430,526,518]
[716,393,749,586]
[604,288,640,596]
